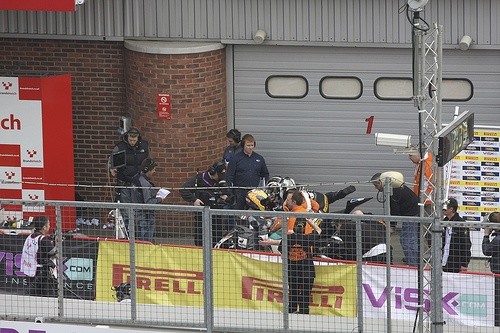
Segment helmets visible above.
[280,176,296,192]
[244,189,268,211]
[265,177,282,196]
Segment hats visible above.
[440,198,458,210]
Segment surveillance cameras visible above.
[374,133,411,148]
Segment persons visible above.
[367,170,432,268]
[440,198,472,271]
[177,162,232,249]
[20,215,65,297]
[115,281,132,305]
[107,128,154,239]
[277,217,322,314]
[482,212,499,327]
[130,158,165,241]
[215,176,394,261]
[223,128,245,210]
[226,133,270,228]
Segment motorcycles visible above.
[214,188,377,262]
